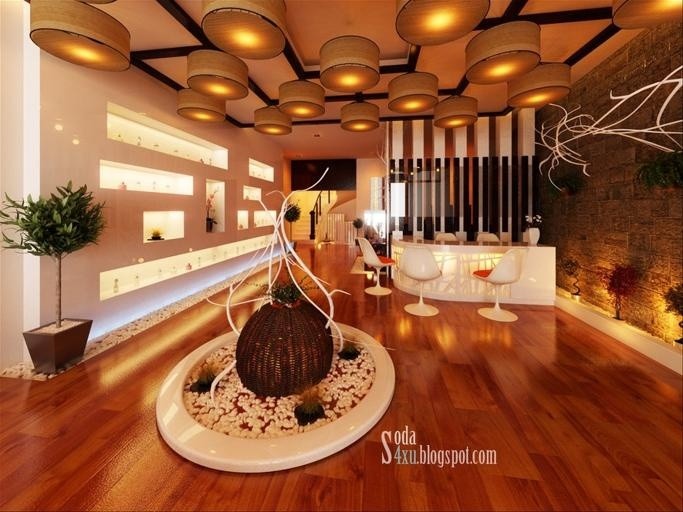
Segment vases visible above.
[528,227,540,247]
[206,220,213,232]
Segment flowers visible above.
[206,188,220,224]
[524,214,543,228]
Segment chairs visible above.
[399,246,442,316]
[356,237,395,296]
[471,246,529,323]
[476,233,499,242]
[435,232,457,241]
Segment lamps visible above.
[29,1,682,137]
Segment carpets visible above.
[349,252,388,277]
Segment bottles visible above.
[117,183,126,190]
[186,263,191,270]
[112,278,118,294]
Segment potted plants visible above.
[284,204,301,253]
[545,161,583,202]
[188,364,219,393]
[293,388,326,428]
[628,145,682,195]
[233,270,333,397]
[352,218,363,246]
[337,332,359,361]
[0,178,112,375]
[150,228,161,240]
